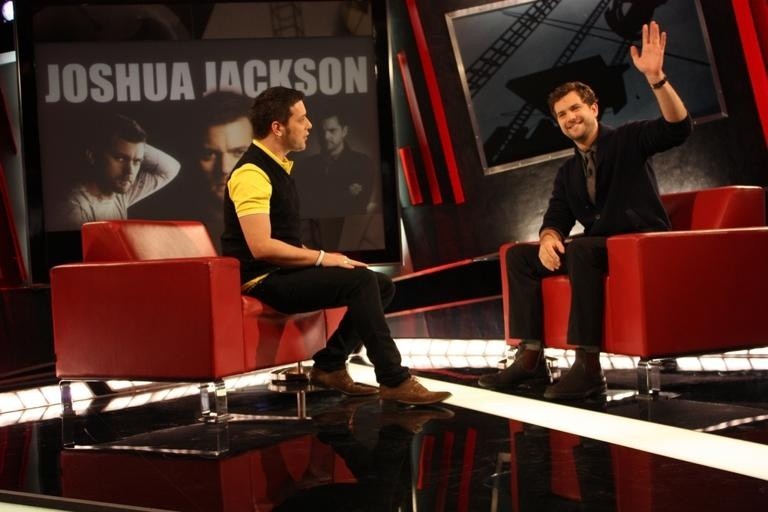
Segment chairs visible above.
[59,415,359,512]
[503,419,767,511]
[49,221,328,460]
[498,185,768,402]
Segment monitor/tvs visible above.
[15,0,403,283]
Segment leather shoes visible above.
[310,396,378,429]
[380,404,455,434]
[379,377,451,404]
[310,367,378,396]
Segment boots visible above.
[477,345,554,390]
[543,348,608,399]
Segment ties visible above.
[585,152,596,205]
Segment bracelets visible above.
[649,75,670,92]
[315,250,325,266]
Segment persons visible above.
[476,20,691,401]
[162,88,256,253]
[300,111,375,218]
[48,114,180,232]
[220,87,451,406]
